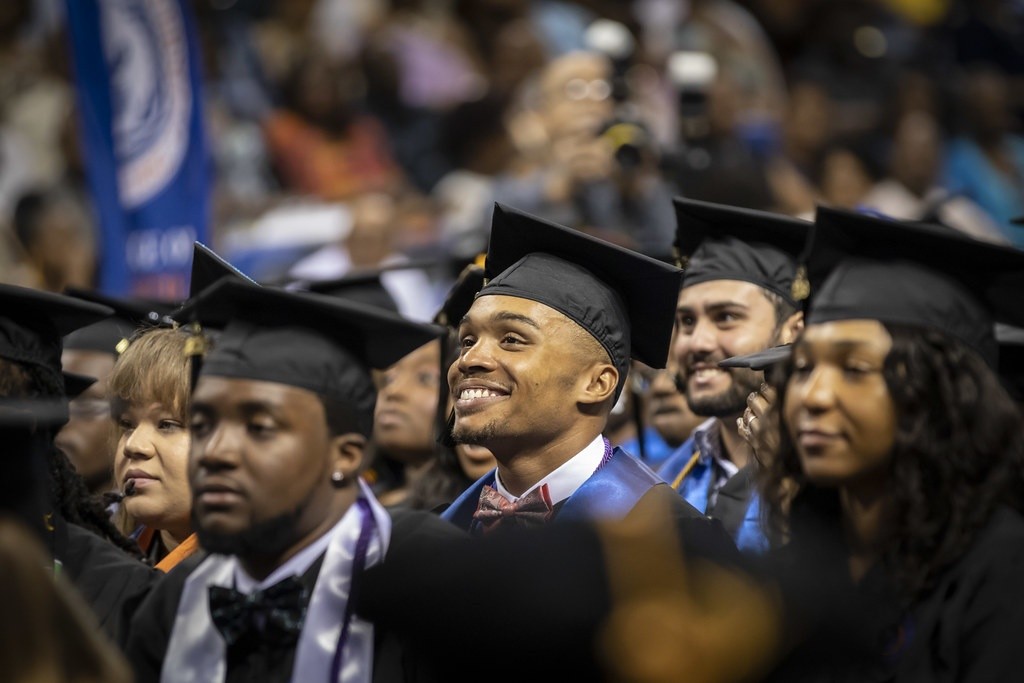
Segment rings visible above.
[748,416,757,427]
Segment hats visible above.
[805,205,1024,369]
[61,288,152,352]
[0,401,70,514]
[718,342,790,386]
[0,283,114,372]
[476,199,685,413]
[168,240,446,412]
[671,197,814,301]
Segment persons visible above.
[0,0,1024,683]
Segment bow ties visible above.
[473,483,553,534]
[206,551,325,645]
